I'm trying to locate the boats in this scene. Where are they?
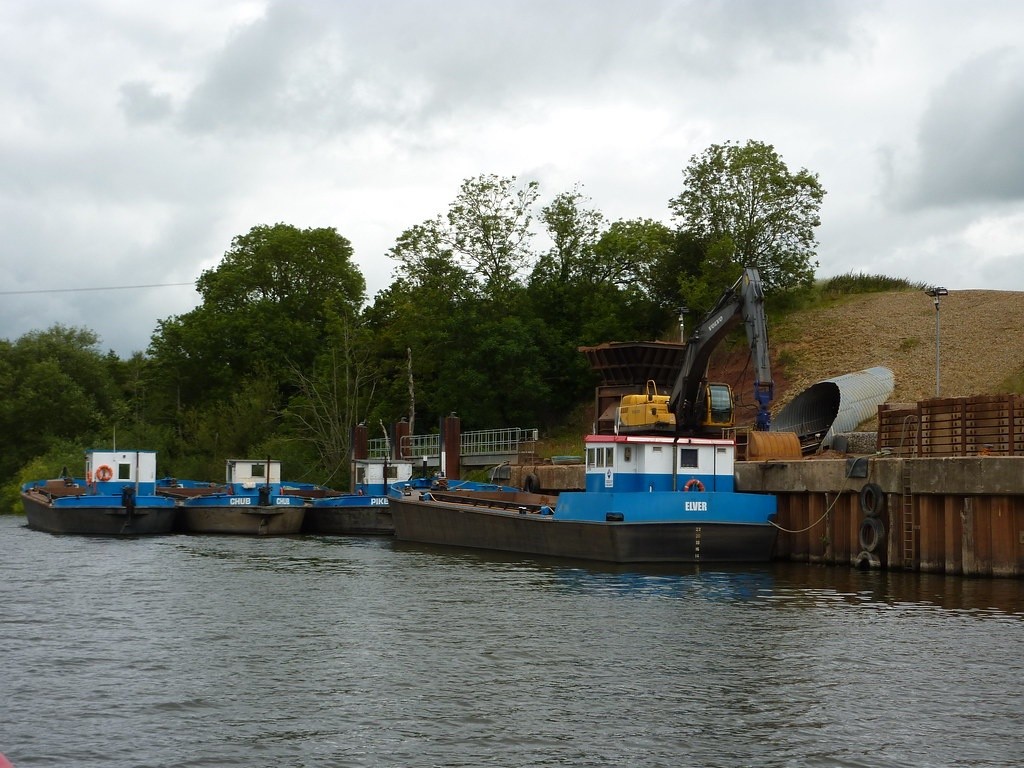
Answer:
[153,479,309,538]
[255,480,397,535]
[386,478,778,567]
[21,476,175,536]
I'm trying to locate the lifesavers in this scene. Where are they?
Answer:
[684,480,704,492]
[96,464,113,481]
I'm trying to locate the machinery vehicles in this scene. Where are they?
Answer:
[614,267,803,463]
